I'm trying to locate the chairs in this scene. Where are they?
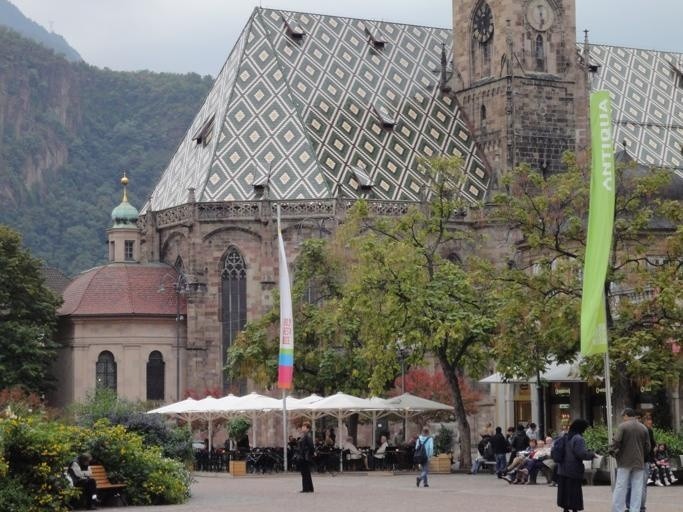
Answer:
[187,447,417,475]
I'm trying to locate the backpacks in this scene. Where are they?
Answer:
[413,436,432,464]
[551,433,567,464]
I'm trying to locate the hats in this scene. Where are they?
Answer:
[78,452,92,467]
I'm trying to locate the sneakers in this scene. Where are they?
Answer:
[416,477,420,487]
[424,484,429,487]
[86,505,97,510]
[655,479,664,487]
[300,489,314,493]
[496,468,538,485]
[647,479,654,484]
[91,499,102,504]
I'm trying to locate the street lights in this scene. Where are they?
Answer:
[529,319,546,440]
[159,269,206,404]
[395,332,408,441]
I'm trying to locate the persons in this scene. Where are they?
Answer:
[475,421,555,486]
[66,453,97,511]
[608,407,678,512]
[555,419,596,511]
[413,427,434,488]
[297,422,313,494]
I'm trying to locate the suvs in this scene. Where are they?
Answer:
[191,438,213,452]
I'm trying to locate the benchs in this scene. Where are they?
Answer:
[614,454,682,483]
[473,457,497,473]
[545,469,597,486]
[62,465,128,506]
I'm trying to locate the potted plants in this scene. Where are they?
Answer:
[427,425,455,475]
[226,415,253,477]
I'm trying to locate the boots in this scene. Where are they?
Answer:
[664,477,671,486]
[670,475,678,483]
